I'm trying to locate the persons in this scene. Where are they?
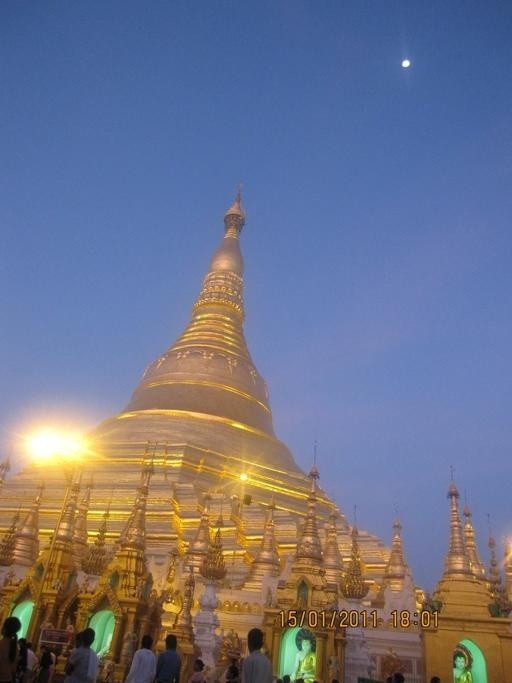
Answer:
[0,616,22,683]
[291,635,317,683]
[48,648,57,683]
[124,635,158,683]
[453,652,473,683]
[224,657,239,683]
[32,645,54,683]
[23,641,39,683]
[64,631,83,675]
[156,634,181,683]
[15,638,28,683]
[187,659,205,683]
[241,628,273,683]
[63,628,97,683]
[276,672,441,683]
